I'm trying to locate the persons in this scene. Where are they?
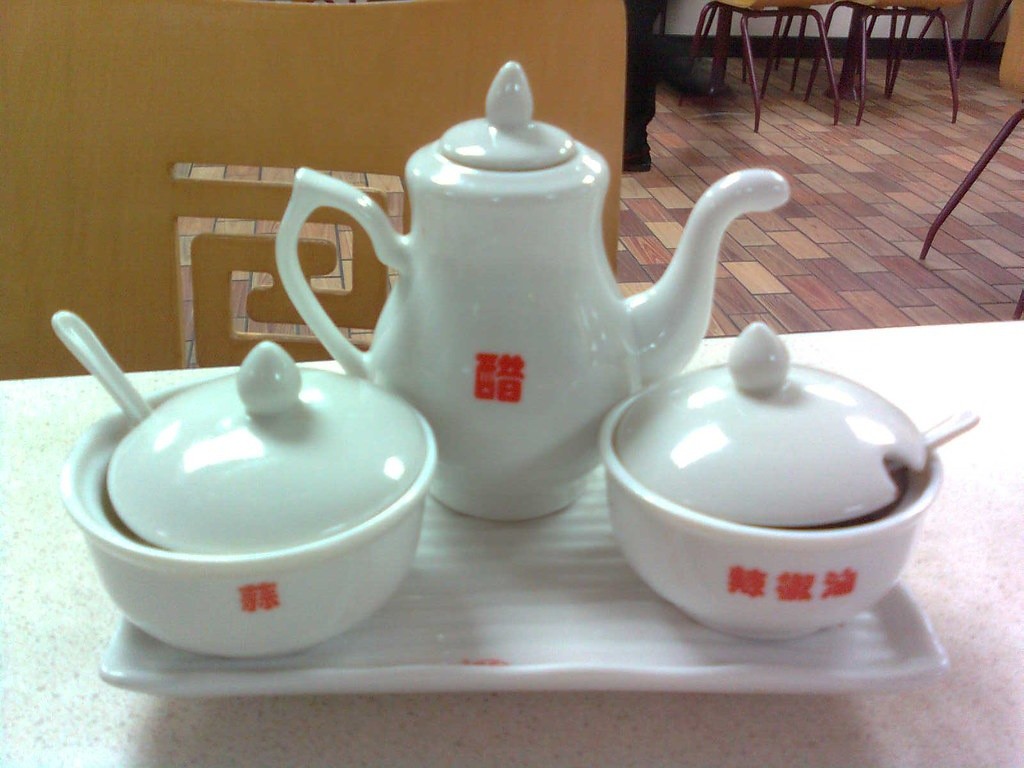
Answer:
[621,0,667,172]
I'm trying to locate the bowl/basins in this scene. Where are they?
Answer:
[62,336,435,663]
[597,322,942,638]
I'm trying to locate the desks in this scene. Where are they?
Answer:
[0,317,1024,768]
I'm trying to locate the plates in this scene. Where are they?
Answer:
[99,468,949,695]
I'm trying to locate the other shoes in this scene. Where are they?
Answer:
[622,153,651,172]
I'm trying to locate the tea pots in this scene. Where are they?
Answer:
[272,60,788,520]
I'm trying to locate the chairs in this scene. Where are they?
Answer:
[802,0,973,126]
[678,0,841,132]
